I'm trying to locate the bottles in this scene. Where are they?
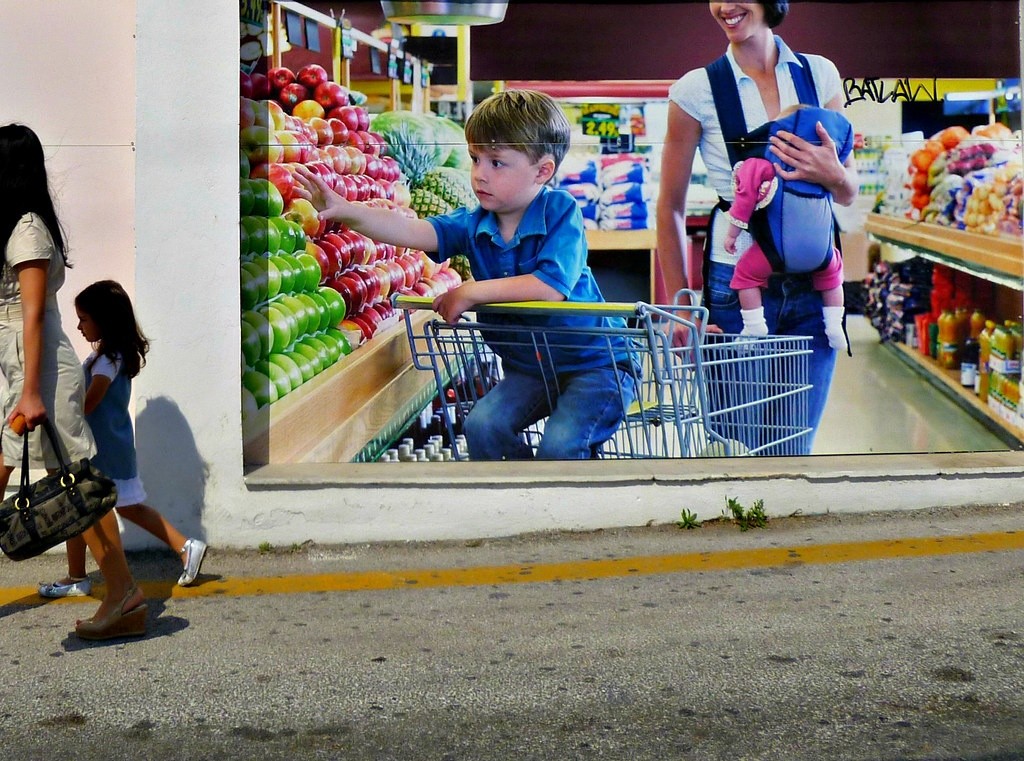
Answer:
[881,136,894,151]
[937,305,1024,430]
[375,369,497,461]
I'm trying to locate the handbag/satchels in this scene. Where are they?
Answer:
[1,418,118,562]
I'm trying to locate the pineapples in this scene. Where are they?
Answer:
[382,122,480,282]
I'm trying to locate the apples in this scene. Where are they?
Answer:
[240,65,462,417]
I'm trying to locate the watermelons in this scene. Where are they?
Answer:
[369,110,472,168]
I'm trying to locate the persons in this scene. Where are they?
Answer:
[0,126,151,640]
[292,90,642,460]
[37,281,207,596]
[725,106,848,350]
[655,1,860,457]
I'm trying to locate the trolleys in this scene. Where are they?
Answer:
[387,286,814,462]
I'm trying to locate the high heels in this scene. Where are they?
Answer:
[74,584,148,640]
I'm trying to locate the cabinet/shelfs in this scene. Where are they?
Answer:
[864,211,1024,450]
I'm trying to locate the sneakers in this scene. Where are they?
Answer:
[177,538,208,586]
[38,575,92,597]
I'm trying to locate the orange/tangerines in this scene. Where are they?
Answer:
[908,123,1009,208]
